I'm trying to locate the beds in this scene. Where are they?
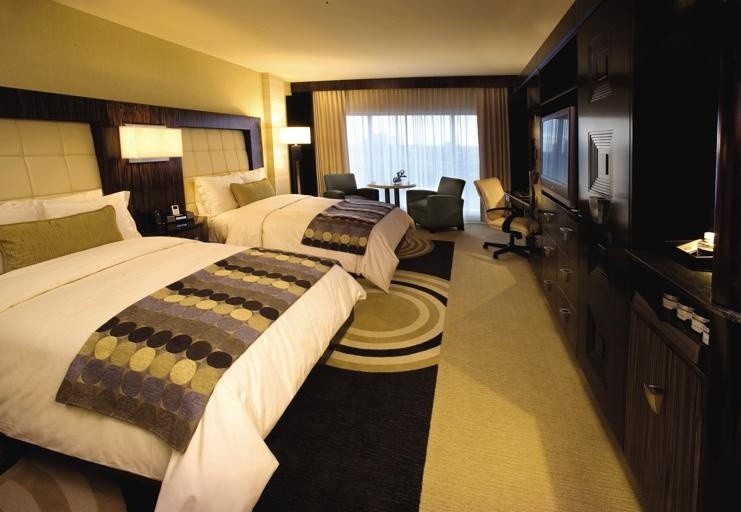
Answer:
[197,195,409,279]
[0,235,356,501]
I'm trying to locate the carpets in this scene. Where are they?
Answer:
[0,235,455,512]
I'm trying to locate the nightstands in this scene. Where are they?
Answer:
[137,211,208,243]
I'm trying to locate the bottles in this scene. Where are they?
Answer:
[696,232,714,255]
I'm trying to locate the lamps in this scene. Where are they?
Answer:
[276,126,311,193]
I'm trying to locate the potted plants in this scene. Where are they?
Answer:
[392,168,406,186]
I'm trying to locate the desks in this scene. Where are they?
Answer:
[504,189,529,216]
[366,181,415,209]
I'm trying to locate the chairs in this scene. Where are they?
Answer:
[405,176,466,233]
[321,173,378,203]
[473,176,530,263]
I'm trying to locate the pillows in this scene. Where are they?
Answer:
[193,166,275,217]
[0,189,142,276]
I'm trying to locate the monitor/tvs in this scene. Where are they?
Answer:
[541,105,577,209]
